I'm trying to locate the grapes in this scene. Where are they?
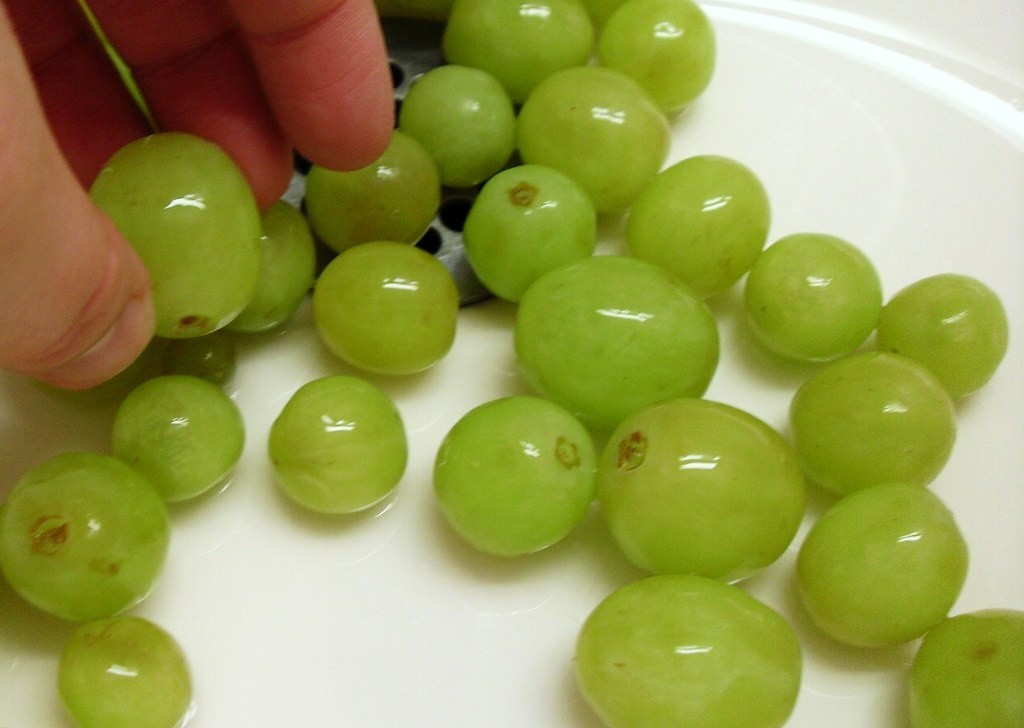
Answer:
[0,0,1024,728]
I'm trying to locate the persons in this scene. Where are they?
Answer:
[0,0,394,393]
